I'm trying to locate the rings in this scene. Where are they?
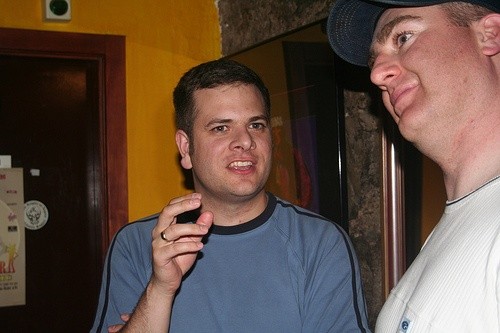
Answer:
[161,232,171,242]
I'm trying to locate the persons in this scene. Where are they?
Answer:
[322,0,500,333]
[89,59,372,333]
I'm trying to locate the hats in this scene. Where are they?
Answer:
[327,0,500,66]
